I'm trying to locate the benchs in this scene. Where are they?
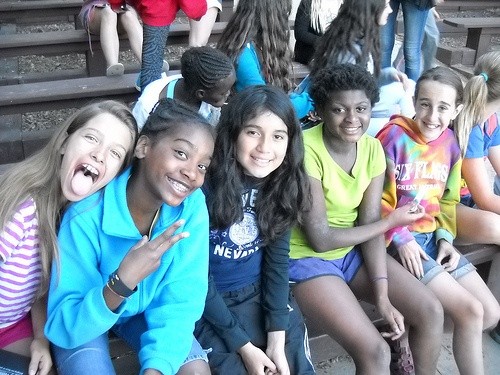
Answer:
[0,0,500,375]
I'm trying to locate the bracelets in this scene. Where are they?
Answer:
[105,270,138,300]
[370,275,390,284]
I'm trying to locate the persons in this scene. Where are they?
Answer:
[372,66,500,375]
[0,100,139,375]
[42,96,212,375]
[447,50,500,345]
[76,0,442,139]
[193,85,317,375]
[288,63,445,375]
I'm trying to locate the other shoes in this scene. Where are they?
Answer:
[105,62,125,76]
[134,71,142,92]
[391,346,415,375]
[161,60,170,73]
